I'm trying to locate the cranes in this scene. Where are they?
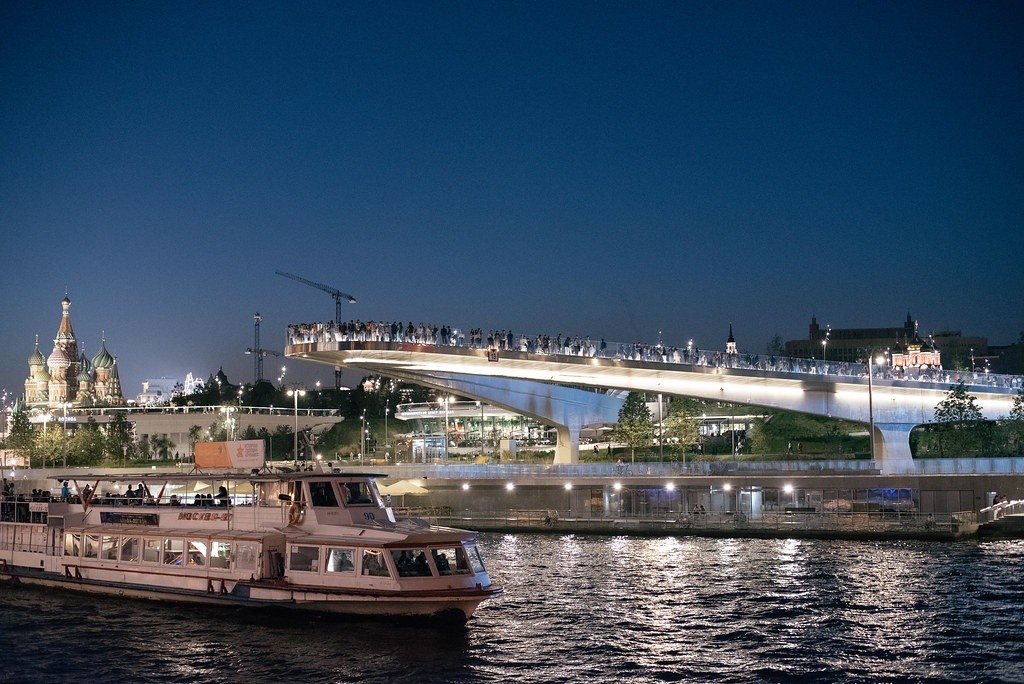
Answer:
[274,268,358,391]
[247,346,281,382]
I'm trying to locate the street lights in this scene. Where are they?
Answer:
[822,341,826,362]
[505,483,514,523]
[438,396,455,462]
[666,483,674,519]
[287,389,306,460]
[564,483,572,518]
[724,484,731,515]
[614,483,622,520]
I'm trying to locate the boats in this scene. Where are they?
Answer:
[0,436,503,633]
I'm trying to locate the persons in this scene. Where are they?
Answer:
[993,495,1008,520]
[166,551,230,568]
[544,511,559,527]
[0,478,231,506]
[693,503,706,521]
[928,512,935,522]
[616,458,624,466]
[287,320,1024,389]
[294,460,341,473]
[593,444,611,457]
[336,547,450,576]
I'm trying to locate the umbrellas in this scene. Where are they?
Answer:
[360,479,434,507]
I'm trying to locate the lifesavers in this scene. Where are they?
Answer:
[289,504,301,525]
